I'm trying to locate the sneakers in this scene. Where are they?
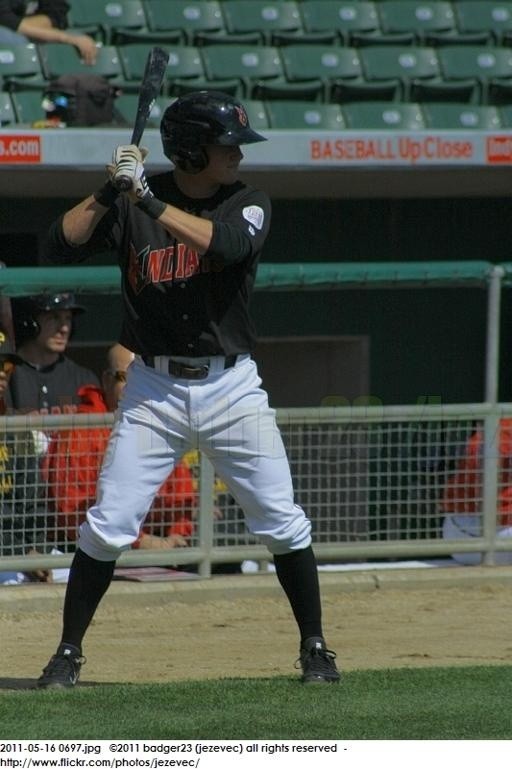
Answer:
[36,642,87,688]
[294,637,341,683]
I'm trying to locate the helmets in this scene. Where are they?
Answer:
[160,90,268,175]
[13,291,87,340]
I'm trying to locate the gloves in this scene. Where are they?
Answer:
[106,144,155,210]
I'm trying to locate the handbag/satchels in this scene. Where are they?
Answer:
[46,72,128,128]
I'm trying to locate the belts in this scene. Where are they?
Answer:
[142,351,237,380]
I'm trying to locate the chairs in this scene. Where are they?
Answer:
[0,0,511,128]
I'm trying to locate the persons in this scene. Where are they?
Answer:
[0,0,99,67]
[0,331,74,584]
[6,292,100,456]
[40,91,339,690]
[44,342,241,573]
[443,419,510,564]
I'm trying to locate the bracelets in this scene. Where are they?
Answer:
[140,196,166,219]
[91,178,118,205]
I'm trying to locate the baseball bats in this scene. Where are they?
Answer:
[114,49,169,191]
